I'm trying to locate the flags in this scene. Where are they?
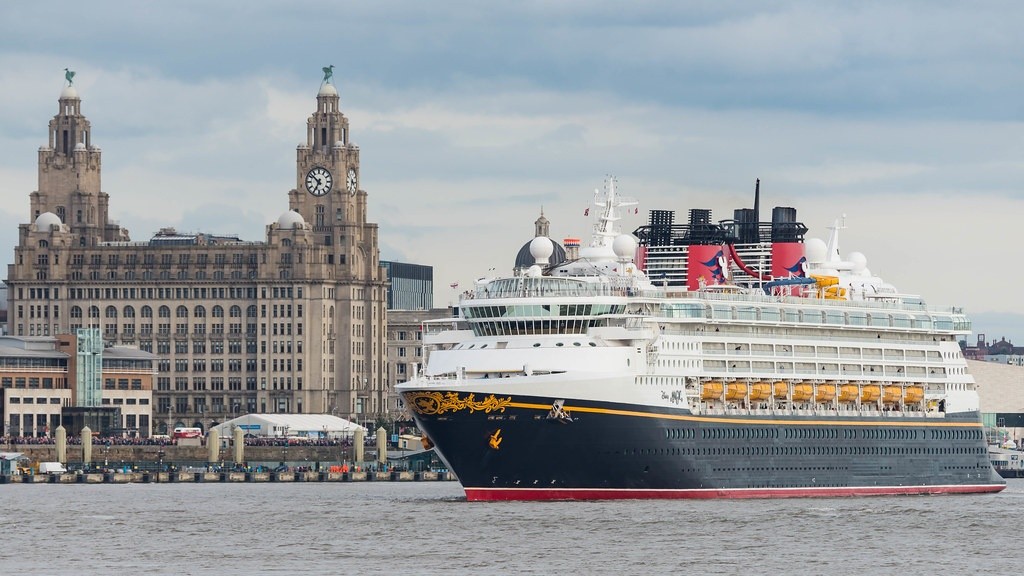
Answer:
[635,207,638,214]
[584,208,589,216]
[420,324,423,330]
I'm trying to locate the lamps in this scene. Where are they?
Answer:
[612,234,636,259]
[804,237,827,267]
[530,235,553,266]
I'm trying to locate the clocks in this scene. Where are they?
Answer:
[345,168,358,197]
[304,167,332,197]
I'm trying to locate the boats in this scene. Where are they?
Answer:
[774,382,788,399]
[883,386,901,404]
[725,382,747,400]
[699,380,723,399]
[792,382,814,400]
[814,385,835,402]
[903,387,923,404]
[861,386,880,402]
[750,382,771,400]
[838,386,859,402]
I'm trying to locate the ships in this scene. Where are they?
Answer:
[393,173,1010,498]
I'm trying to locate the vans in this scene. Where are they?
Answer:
[39,462,68,475]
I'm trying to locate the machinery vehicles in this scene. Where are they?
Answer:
[16,457,33,475]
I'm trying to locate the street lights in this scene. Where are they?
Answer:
[342,427,348,465]
[166,405,173,435]
[233,404,238,428]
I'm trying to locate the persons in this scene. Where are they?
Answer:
[0,435,177,446]
[208,463,263,472]
[363,438,377,447]
[229,435,354,447]
[297,464,412,474]
